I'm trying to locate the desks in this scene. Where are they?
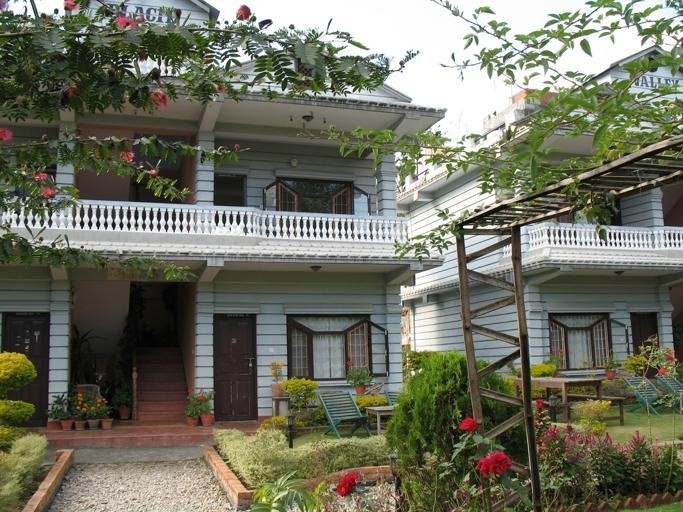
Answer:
[510,373,607,426]
[361,405,395,434]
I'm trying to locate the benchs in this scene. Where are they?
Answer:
[533,397,568,424]
[552,391,625,428]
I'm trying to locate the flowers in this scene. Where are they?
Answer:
[266,360,286,384]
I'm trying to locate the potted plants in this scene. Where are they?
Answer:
[602,350,618,381]
[344,366,374,396]
[196,387,216,427]
[182,391,199,427]
[41,375,132,431]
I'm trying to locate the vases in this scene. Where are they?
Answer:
[269,382,284,397]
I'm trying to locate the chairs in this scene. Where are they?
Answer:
[621,375,680,416]
[316,390,373,443]
[653,373,683,415]
[383,388,402,406]
